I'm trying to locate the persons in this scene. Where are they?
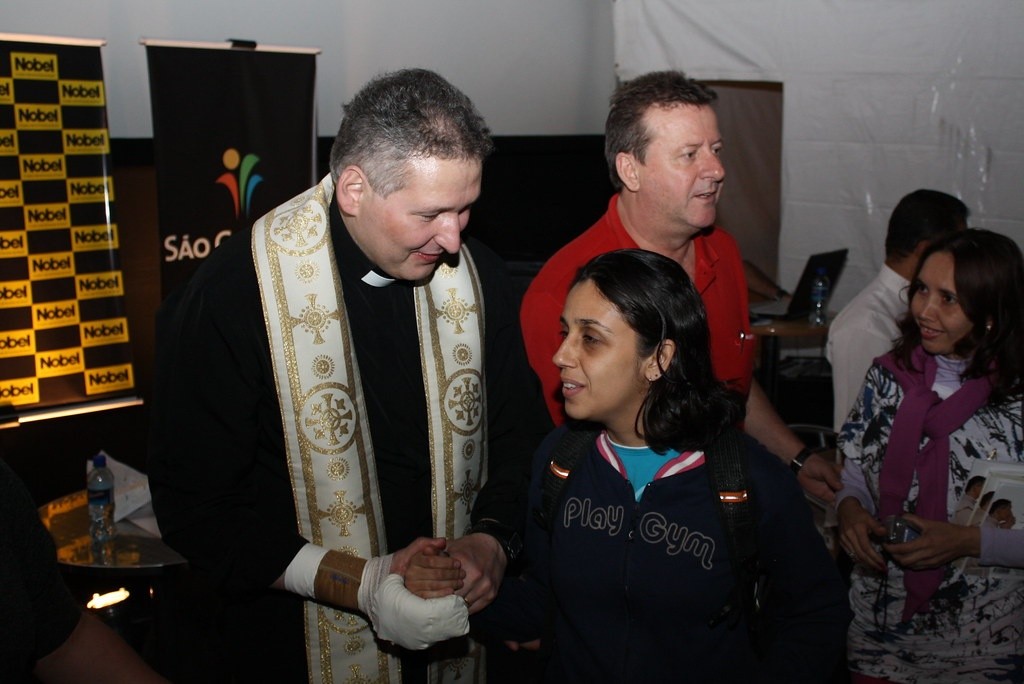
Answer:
[985,498,1017,531]
[741,259,791,318]
[404,247,838,683]
[155,62,541,684]
[972,490,995,528]
[0,459,170,684]
[824,188,971,433]
[830,228,1024,683]
[955,476,985,527]
[518,66,845,506]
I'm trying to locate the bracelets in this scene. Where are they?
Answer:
[773,290,785,301]
[790,448,813,476]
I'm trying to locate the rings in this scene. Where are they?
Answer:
[463,597,471,606]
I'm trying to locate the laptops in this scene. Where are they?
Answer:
[749,248,849,320]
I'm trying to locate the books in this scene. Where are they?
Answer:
[946,460,1024,582]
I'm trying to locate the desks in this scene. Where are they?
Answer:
[36,493,191,621]
[752,310,839,408]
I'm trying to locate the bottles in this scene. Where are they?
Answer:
[85,455,118,540]
[808,269,831,326]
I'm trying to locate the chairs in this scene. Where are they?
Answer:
[787,423,842,564]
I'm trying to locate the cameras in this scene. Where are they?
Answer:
[886,513,921,545]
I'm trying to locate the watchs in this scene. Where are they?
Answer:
[467,519,524,559]
